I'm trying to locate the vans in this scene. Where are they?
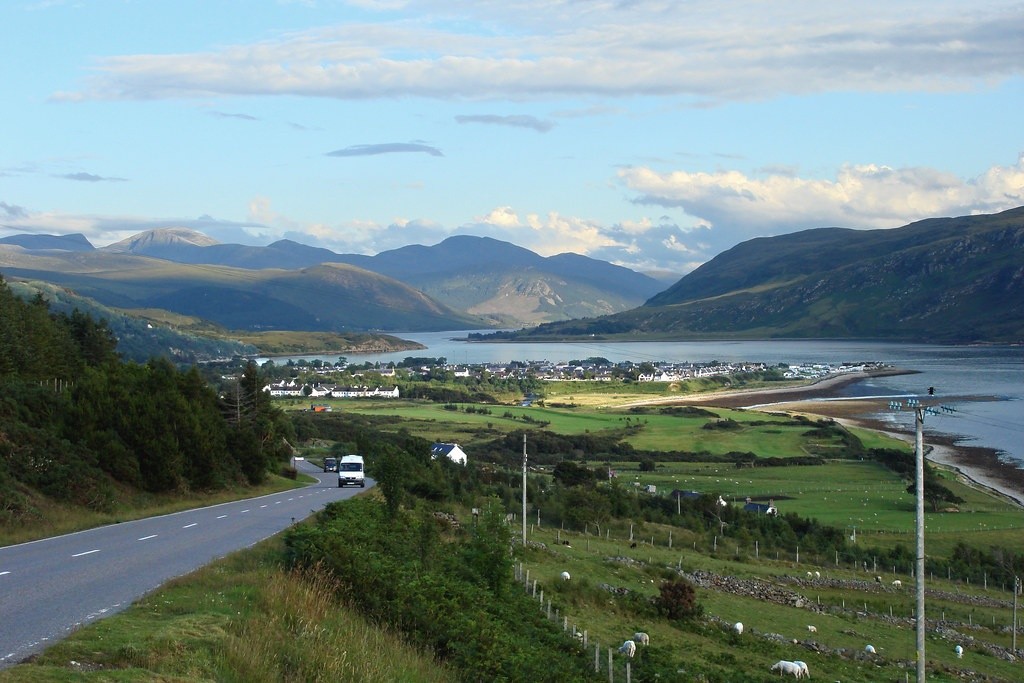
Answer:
[324,458,337,473]
[337,454,366,488]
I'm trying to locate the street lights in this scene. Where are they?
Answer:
[884,399,958,683]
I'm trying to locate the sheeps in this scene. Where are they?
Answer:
[561,544,963,681]
